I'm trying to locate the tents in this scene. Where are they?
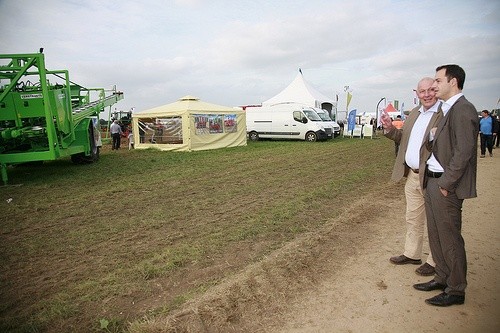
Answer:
[261,68,336,123]
[132,96,246,152]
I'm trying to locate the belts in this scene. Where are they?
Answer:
[426,168,443,178]
[408,165,419,173]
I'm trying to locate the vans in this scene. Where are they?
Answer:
[312,108,340,139]
[245,107,334,142]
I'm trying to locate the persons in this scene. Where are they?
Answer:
[138,119,164,143]
[479,110,500,157]
[110,120,124,152]
[380,77,445,277]
[394,115,403,158]
[412,64,480,308]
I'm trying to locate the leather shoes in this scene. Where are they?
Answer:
[390,254,422,265]
[413,279,448,292]
[415,262,436,276]
[425,292,465,307]
